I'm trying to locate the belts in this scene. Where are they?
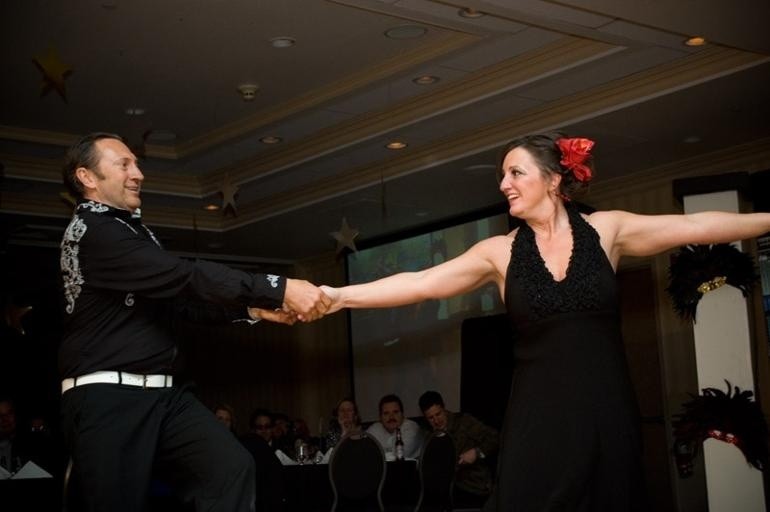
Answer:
[62,371,173,394]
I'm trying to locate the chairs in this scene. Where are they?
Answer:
[326,431,458,512]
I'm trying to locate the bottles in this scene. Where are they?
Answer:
[395,428,405,461]
